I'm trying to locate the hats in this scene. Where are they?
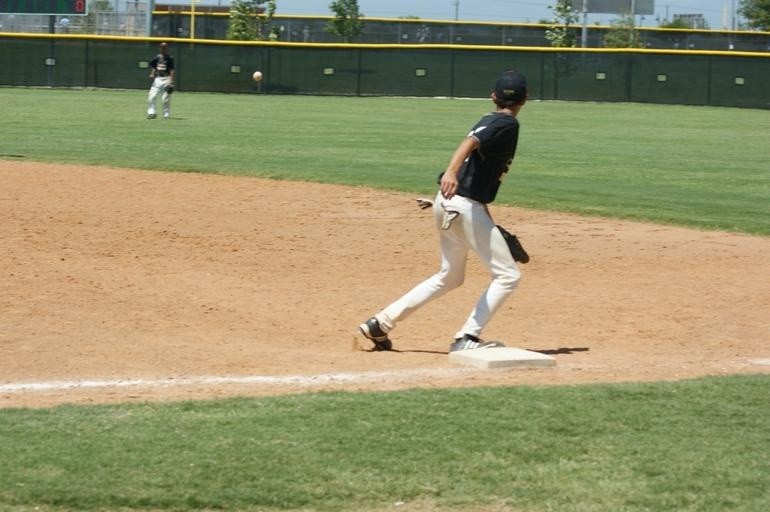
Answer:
[494,69,526,101]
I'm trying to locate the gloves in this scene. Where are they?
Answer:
[416,199,433,210]
[441,211,458,230]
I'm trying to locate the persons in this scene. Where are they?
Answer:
[357,71,528,352]
[146,42,175,119]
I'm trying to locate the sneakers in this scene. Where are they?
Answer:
[146,114,155,119]
[359,317,392,351]
[449,333,504,353]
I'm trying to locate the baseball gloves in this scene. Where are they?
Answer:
[495,223,529,264]
[164,81,175,92]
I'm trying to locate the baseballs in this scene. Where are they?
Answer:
[253,72,263,82]
